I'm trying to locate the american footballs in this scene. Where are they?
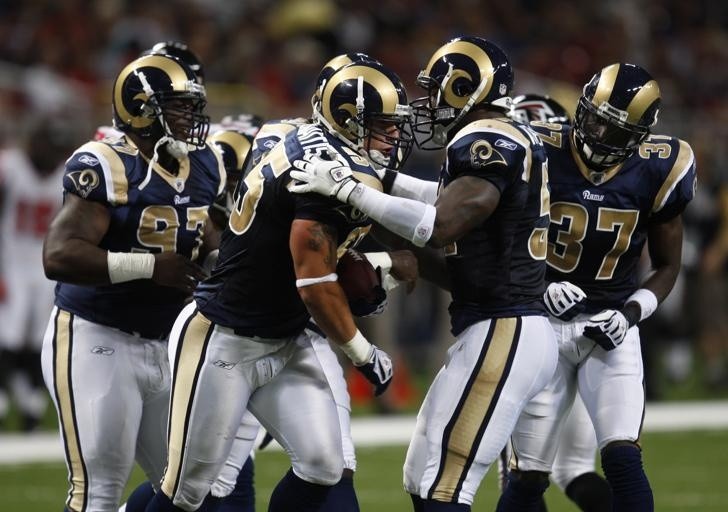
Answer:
[336,247,380,307]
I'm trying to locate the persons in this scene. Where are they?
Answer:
[288,35,698,512]
[35,42,416,512]
[0,1,727,418]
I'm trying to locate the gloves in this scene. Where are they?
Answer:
[582,308,629,351]
[351,343,393,398]
[542,281,587,321]
[288,144,360,204]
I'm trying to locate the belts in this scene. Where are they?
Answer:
[119,329,168,340]
[233,321,326,339]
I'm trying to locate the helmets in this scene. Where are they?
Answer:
[152,40,205,85]
[406,35,514,150]
[112,53,209,147]
[311,51,378,120]
[312,60,415,173]
[220,112,264,138]
[571,61,661,171]
[507,93,571,126]
